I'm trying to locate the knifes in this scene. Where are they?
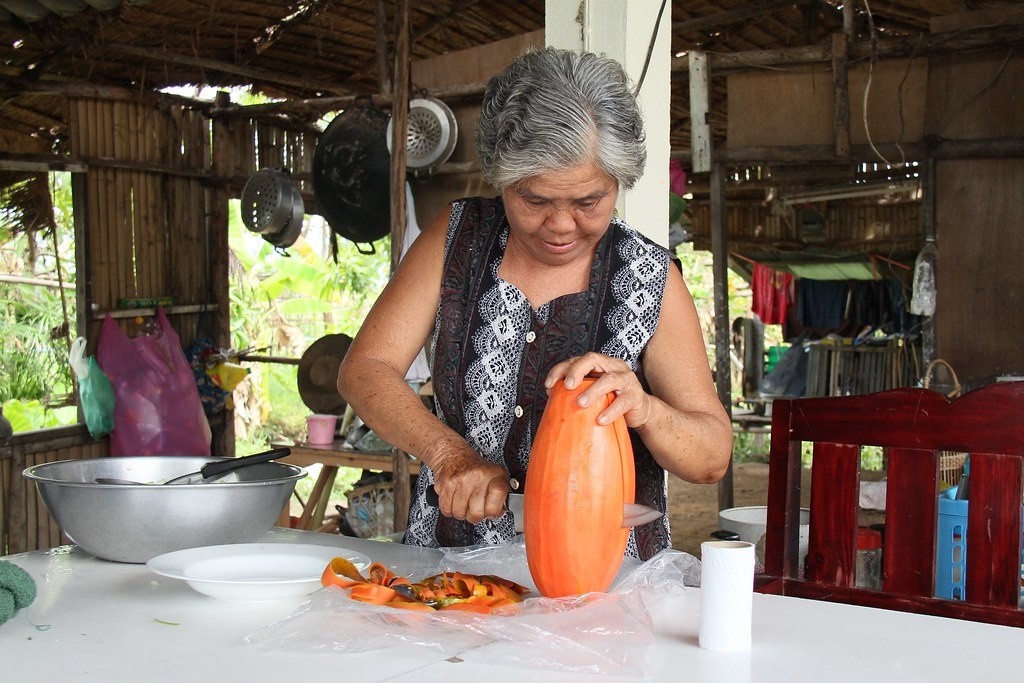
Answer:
[424,483,664,533]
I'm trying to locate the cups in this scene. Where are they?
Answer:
[699,540,757,652]
[303,414,338,446]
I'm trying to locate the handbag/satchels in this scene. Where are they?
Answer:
[67,336,89,382]
[98,303,213,457]
[184,303,233,421]
[70,355,117,439]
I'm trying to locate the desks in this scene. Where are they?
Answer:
[271,439,422,539]
[0,526,1024,683]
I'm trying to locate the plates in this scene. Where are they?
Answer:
[145,542,371,602]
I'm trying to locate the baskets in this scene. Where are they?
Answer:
[925,359,968,489]
[344,482,395,539]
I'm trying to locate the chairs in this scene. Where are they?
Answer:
[757,382,1024,629]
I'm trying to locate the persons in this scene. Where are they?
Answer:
[337,46,733,561]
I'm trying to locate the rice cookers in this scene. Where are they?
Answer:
[710,504,812,573]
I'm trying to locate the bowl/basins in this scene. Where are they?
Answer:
[21,454,307,565]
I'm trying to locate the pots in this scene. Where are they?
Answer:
[313,93,392,256]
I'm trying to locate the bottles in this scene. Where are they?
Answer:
[911,234,937,316]
[135,316,157,334]
[204,361,250,393]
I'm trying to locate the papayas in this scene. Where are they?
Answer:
[522,378,637,601]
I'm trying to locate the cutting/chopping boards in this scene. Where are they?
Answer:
[297,333,353,415]
